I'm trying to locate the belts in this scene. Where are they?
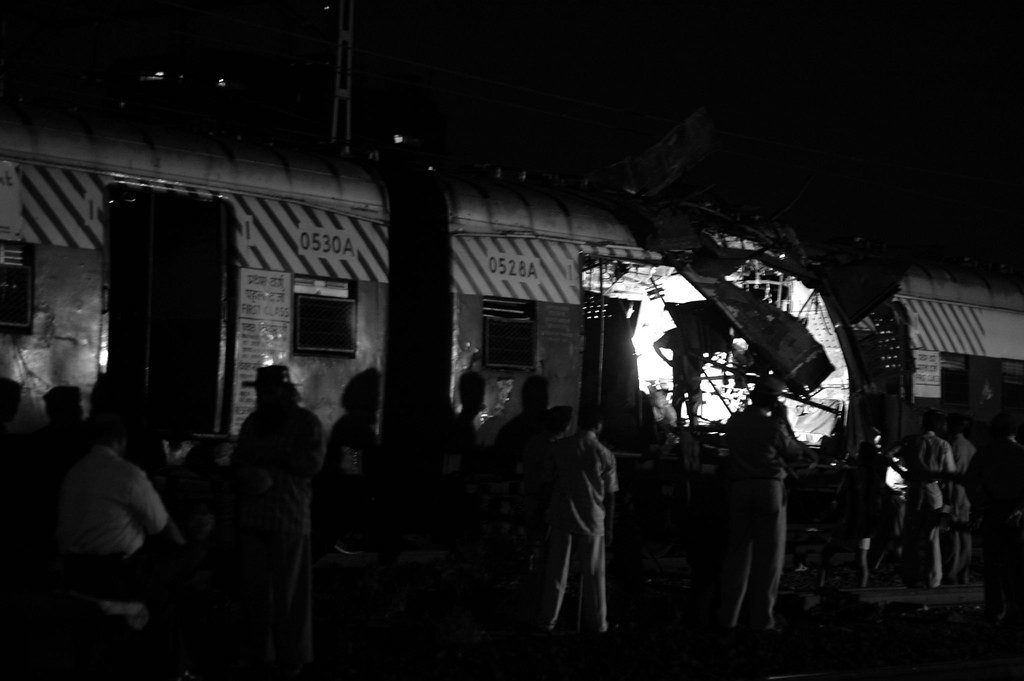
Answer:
[731,476,782,481]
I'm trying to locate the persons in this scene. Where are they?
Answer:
[653,326,702,426]
[538,402,620,633]
[54,365,1023,681]
